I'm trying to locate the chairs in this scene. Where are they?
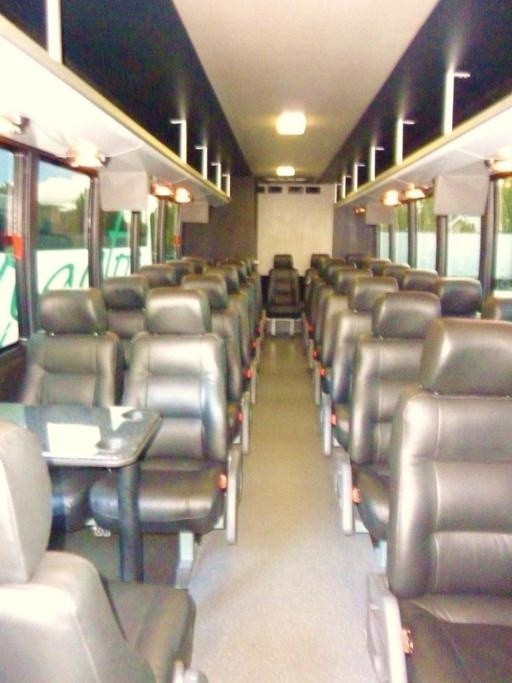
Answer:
[262,253,305,337]
[366,318,512,683]
[0,413,195,683]
[298,252,512,568]
[0,252,262,590]
